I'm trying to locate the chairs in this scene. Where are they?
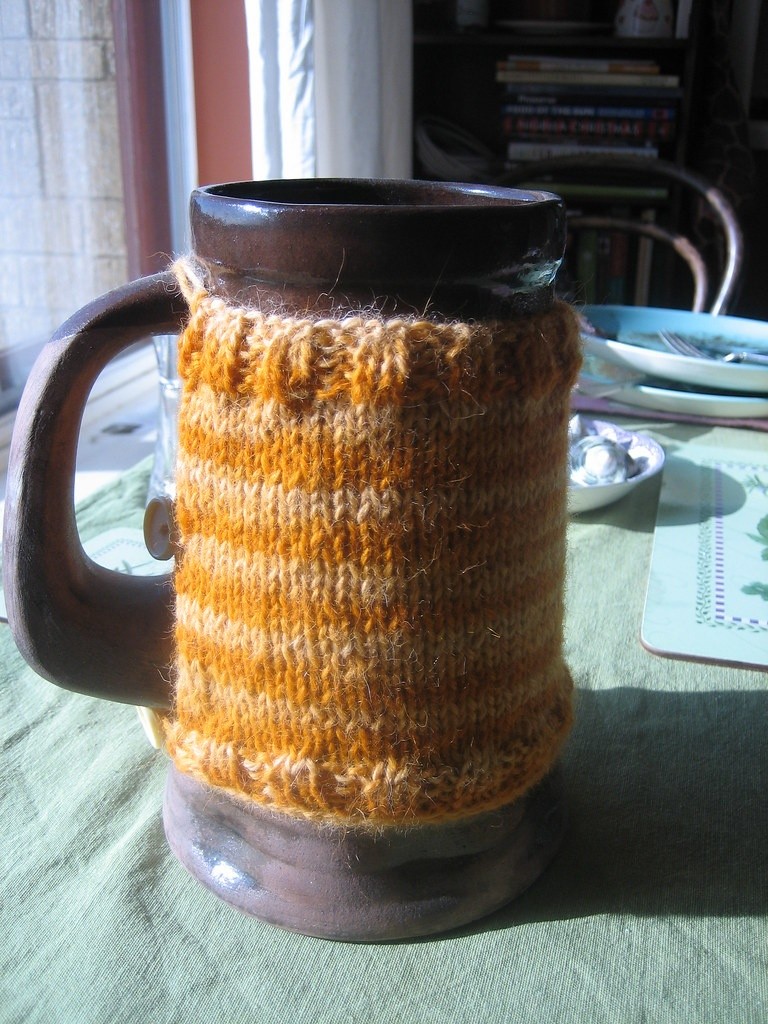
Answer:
[497,152,744,319]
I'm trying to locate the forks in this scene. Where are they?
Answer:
[656,330,768,369]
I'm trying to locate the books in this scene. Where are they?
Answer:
[496,54,684,304]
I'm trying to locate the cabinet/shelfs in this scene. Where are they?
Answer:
[412,30,709,307]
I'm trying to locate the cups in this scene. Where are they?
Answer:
[0,177,575,943]
[520,0,573,21]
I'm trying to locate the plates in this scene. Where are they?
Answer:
[492,20,605,34]
[574,305,768,417]
[569,413,665,513]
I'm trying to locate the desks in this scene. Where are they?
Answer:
[0,351,767,1024]
